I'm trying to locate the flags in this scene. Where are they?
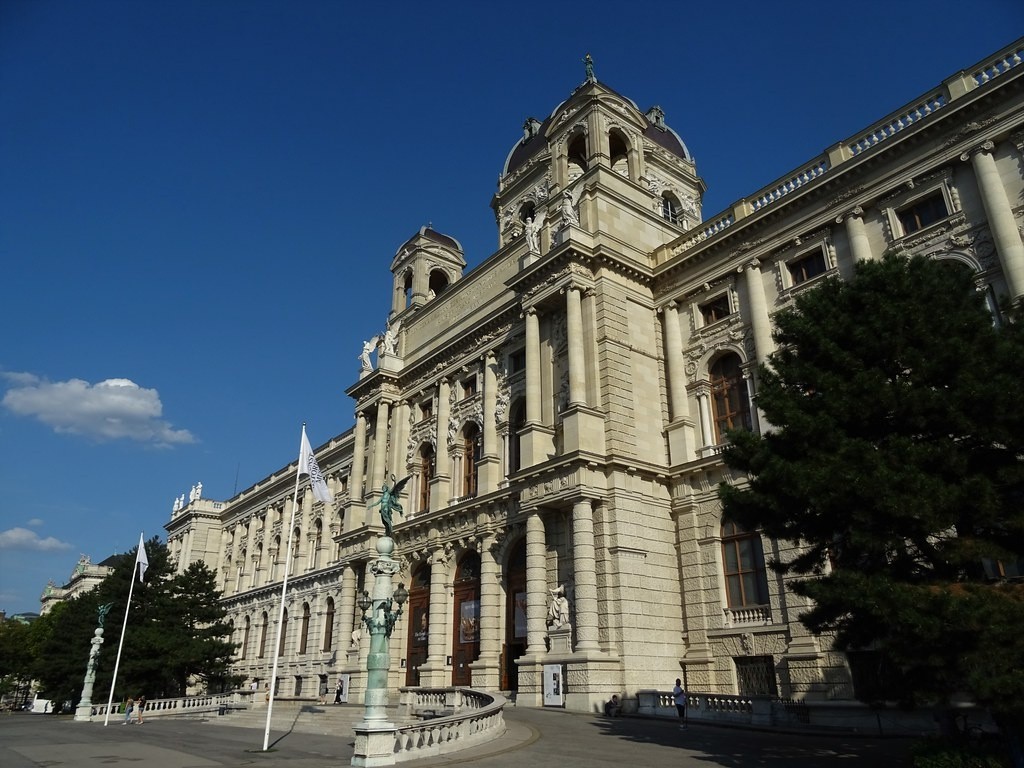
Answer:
[297,426,335,503]
[137,533,149,582]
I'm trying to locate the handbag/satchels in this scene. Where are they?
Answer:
[325,688,328,694]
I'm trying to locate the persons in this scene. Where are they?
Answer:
[189,485,195,503]
[383,323,396,357]
[367,485,394,536]
[173,497,179,513]
[135,695,147,724]
[605,695,618,717]
[673,678,690,729]
[557,189,579,226]
[265,682,269,702]
[251,680,258,690]
[933,703,969,740]
[122,695,134,726]
[195,481,202,499]
[519,212,537,251]
[333,680,343,705]
[318,673,328,706]
[178,494,185,510]
[358,340,373,372]
[550,592,570,627]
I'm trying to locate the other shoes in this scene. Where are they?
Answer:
[129,720,133,723]
[680,725,689,731]
[333,702,335,705]
[135,722,139,724]
[122,723,127,725]
[339,702,342,705]
[139,722,143,724]
